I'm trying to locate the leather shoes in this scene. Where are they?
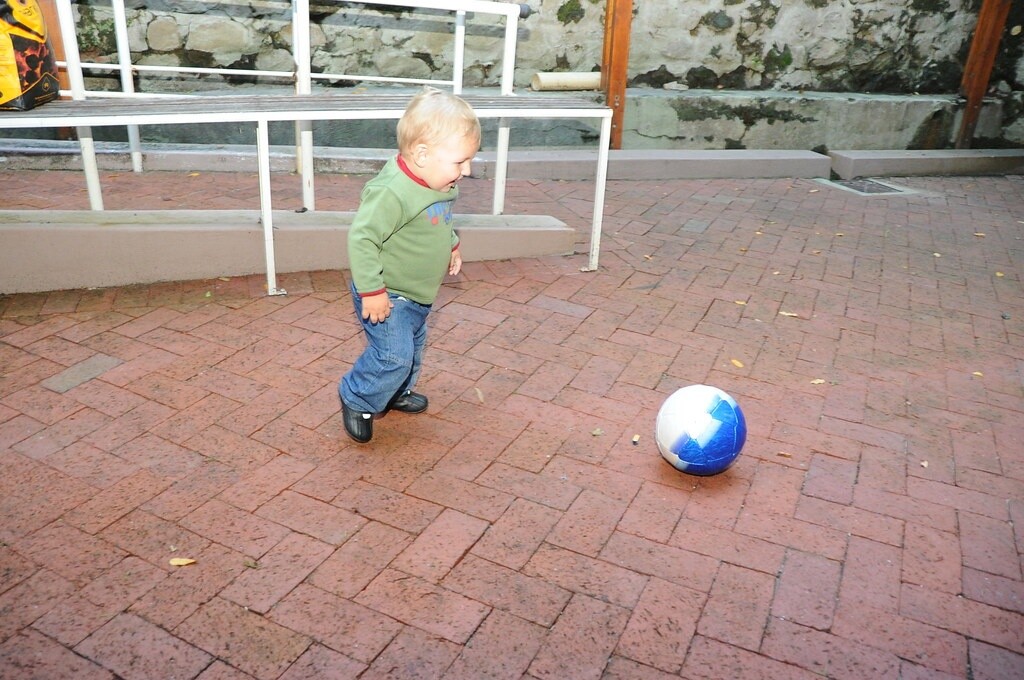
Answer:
[386,390,428,413]
[339,393,372,443]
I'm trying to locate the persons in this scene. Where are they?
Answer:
[339,91,481,443]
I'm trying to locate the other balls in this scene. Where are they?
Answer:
[655,383,746,478]
